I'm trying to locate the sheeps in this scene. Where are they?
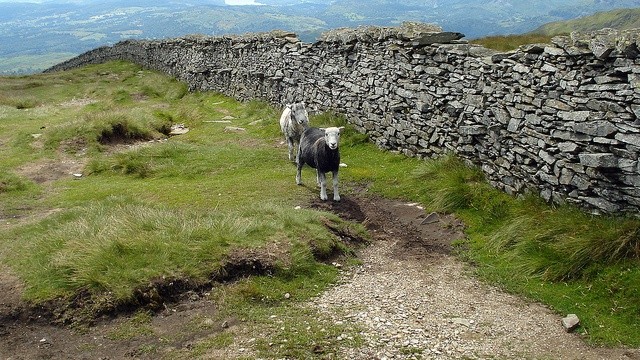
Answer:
[295,125,345,201]
[279,100,309,160]
